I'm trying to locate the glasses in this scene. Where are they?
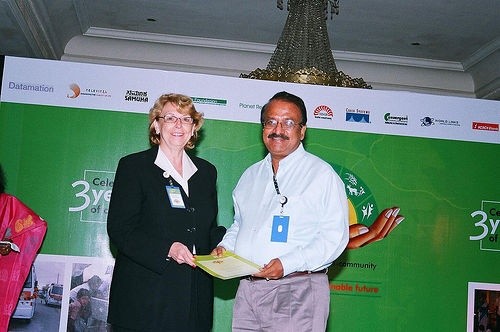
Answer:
[263,119,302,129]
[156,115,195,124]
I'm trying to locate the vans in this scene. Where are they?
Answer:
[38,283,63,307]
[11,264,38,319]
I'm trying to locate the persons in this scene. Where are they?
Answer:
[210,91,350,332]
[67,275,102,332]
[106,92,227,332]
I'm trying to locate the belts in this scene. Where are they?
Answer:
[242,269,326,280]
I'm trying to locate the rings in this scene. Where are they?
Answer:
[266,277,269,281]
